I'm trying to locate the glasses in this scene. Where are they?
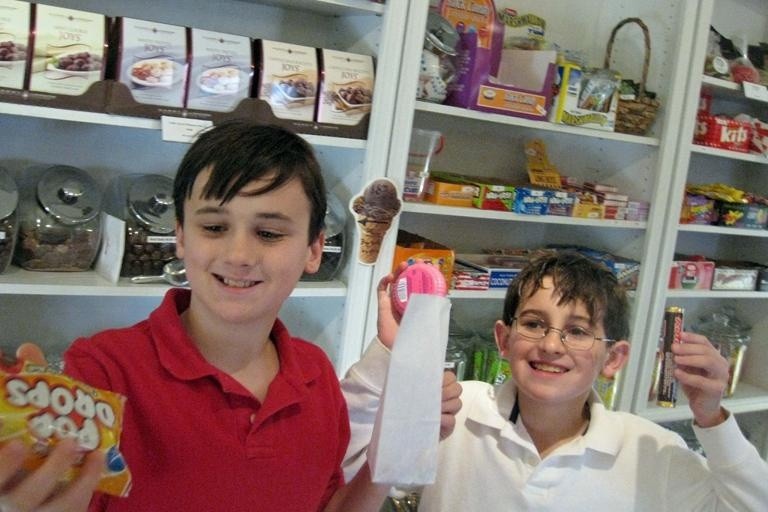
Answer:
[511,314,618,352]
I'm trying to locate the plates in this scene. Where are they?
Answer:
[0,41,254,95]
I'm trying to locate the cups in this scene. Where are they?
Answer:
[402,128,443,203]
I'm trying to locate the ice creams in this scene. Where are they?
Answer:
[350,179,402,264]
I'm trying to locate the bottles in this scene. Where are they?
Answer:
[415,8,463,104]
[689,305,752,399]
[13,163,103,273]
[298,190,347,282]
[105,172,177,278]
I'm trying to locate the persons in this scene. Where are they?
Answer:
[337,257,768,512]
[0,117,462,512]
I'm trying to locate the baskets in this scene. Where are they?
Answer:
[602,17,661,136]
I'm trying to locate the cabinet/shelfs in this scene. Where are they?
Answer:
[1,0,768,509]
